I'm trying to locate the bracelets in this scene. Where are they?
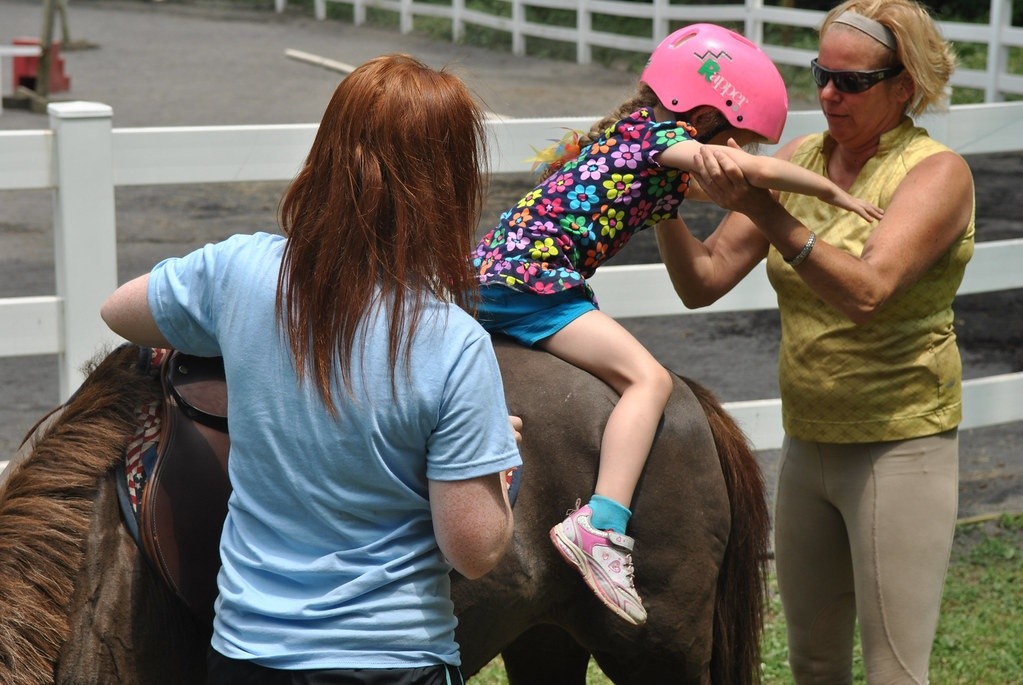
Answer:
[786,229,819,266]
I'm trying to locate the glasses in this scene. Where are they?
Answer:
[810,58,905,93]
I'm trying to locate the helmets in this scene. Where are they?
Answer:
[640,22,789,144]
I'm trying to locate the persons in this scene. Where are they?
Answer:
[654,1,981,685]
[99,51,524,685]
[462,21,886,624]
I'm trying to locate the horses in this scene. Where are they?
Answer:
[0,340,776,685]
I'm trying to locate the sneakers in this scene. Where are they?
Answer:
[548,503,649,626]
